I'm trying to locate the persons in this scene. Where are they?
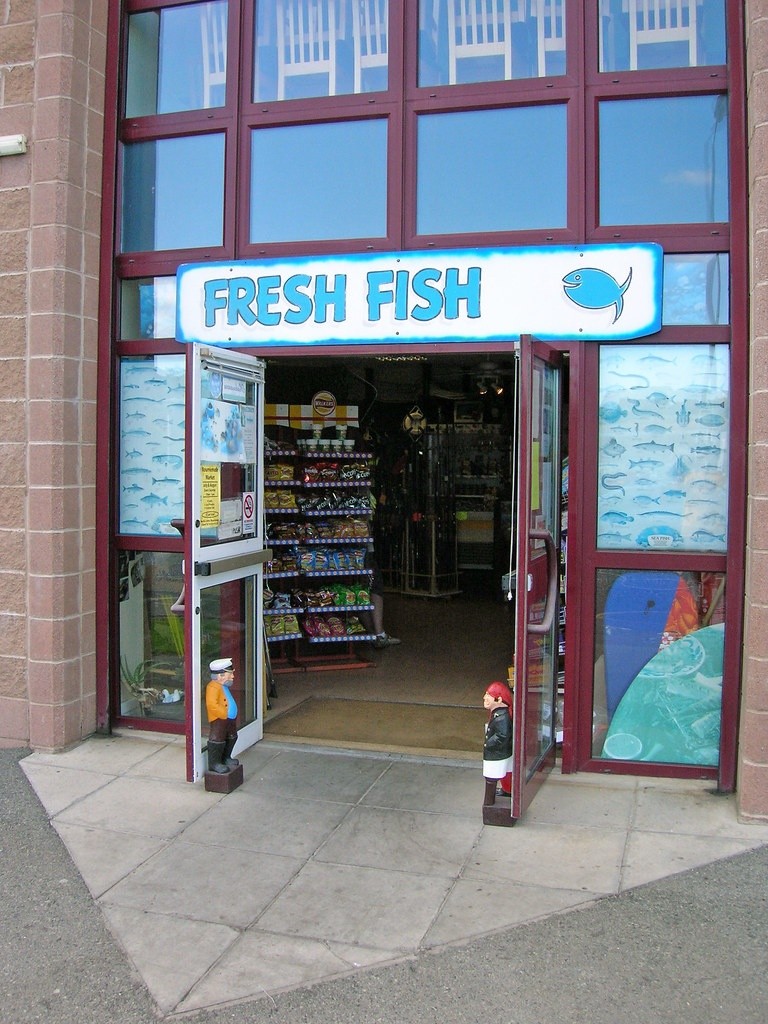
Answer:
[482,681,514,798]
[358,492,401,647]
[206,658,239,773]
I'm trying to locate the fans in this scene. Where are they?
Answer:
[446,354,515,377]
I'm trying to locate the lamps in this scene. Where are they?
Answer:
[492,378,503,394]
[476,378,486,395]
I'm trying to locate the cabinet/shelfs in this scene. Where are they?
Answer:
[299,452,380,642]
[264,449,303,642]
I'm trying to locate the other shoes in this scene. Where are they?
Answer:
[374,631,401,648]
